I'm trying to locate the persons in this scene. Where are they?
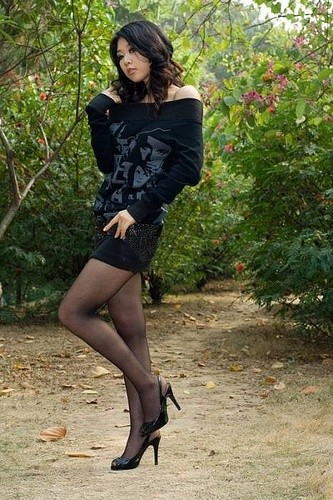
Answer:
[57,21,204,470]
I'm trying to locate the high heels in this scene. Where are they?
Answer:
[110,433,161,470]
[141,375,181,435]
